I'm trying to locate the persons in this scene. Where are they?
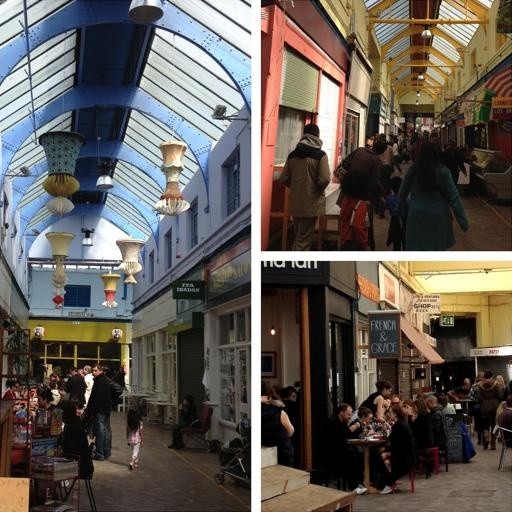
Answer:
[1,363,145,479]
[278,122,485,250]
[167,394,197,449]
[261,369,512,495]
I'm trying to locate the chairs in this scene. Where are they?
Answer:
[497,425,512,471]
[180,406,214,448]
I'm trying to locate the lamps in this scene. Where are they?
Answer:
[5,166,32,177]
[154,32,191,215]
[95,104,114,189]
[128,0,164,23]
[271,287,276,335]
[100,263,121,307]
[116,201,145,284]
[38,30,86,215]
[45,213,76,309]
[80,199,95,246]
[415,22,432,106]
[211,103,249,121]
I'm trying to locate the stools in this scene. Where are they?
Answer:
[65,477,97,512]
[322,468,351,490]
[34,479,68,503]
[392,443,449,494]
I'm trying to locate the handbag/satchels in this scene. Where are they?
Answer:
[333,163,348,179]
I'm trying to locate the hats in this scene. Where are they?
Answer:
[303,123,319,138]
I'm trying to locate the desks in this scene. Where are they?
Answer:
[118,390,177,431]
[0,400,13,476]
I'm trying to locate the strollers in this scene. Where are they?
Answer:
[214,418,251,486]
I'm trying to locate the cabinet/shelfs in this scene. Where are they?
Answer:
[470,147,512,199]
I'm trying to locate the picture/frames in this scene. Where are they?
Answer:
[261,351,277,379]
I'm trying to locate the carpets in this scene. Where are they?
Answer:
[346,436,387,494]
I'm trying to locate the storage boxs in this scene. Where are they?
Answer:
[32,460,79,482]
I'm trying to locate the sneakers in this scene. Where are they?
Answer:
[129,461,141,470]
[355,484,394,497]
[168,441,184,450]
[93,453,111,461]
